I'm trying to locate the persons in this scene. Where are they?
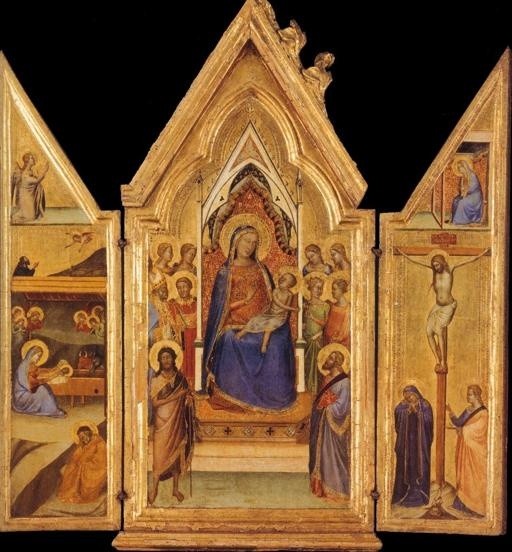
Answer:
[59,425,108,504]
[451,159,484,223]
[395,245,488,374]
[65,231,95,252]
[15,306,105,418]
[147,239,201,394]
[14,255,38,276]
[203,225,297,415]
[234,271,295,354]
[307,349,350,505]
[300,238,351,394]
[11,152,48,223]
[146,349,194,507]
[393,383,435,508]
[445,384,486,519]
